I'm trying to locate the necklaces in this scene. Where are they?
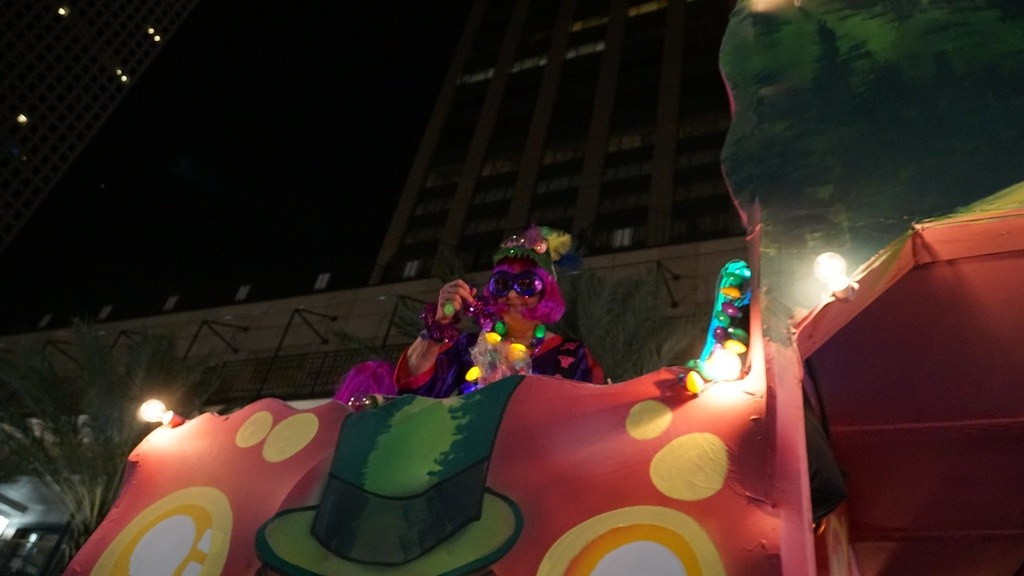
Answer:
[530,336,543,359]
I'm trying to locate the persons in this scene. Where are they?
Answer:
[392,226,610,399]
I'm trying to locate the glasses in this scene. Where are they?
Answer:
[488,270,543,297]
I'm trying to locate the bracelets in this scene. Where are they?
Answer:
[418,304,463,343]
